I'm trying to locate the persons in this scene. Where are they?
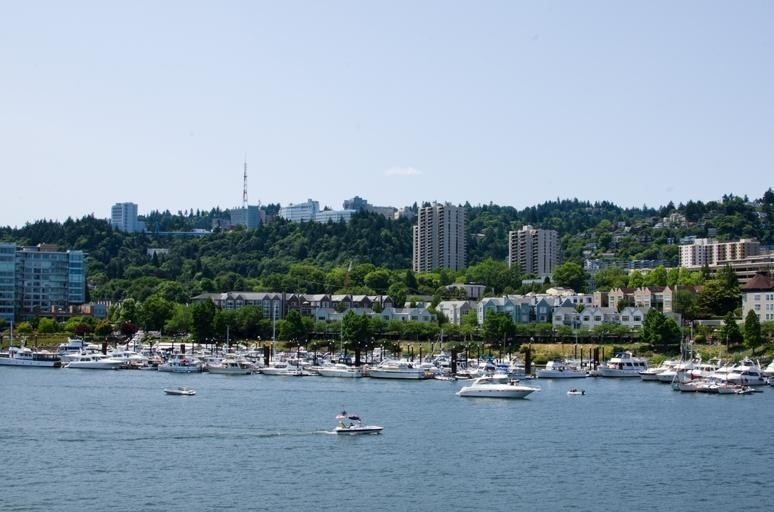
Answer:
[339,421,353,429]
[569,388,578,392]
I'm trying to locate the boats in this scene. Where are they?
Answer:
[304,363,360,378]
[65,343,316,376]
[455,374,540,398]
[164,385,196,396]
[368,353,536,380]
[3,346,62,368]
[637,356,772,393]
[538,360,586,379]
[333,423,385,437]
[596,351,648,377]
